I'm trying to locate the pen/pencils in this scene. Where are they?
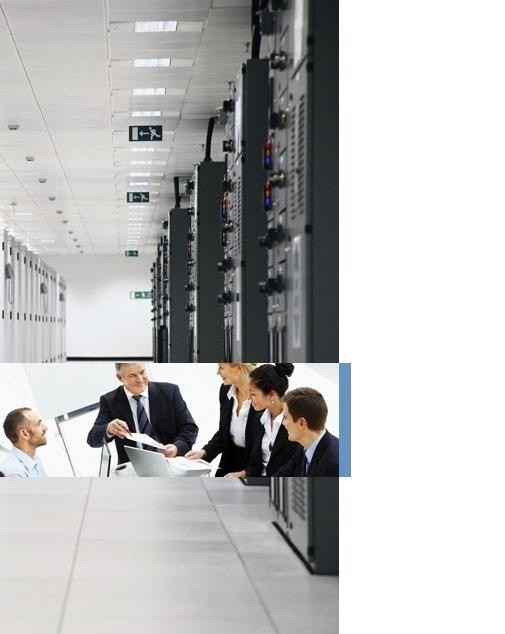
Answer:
[122,426,133,438]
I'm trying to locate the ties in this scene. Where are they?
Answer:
[300,452,309,477]
[131,394,162,452]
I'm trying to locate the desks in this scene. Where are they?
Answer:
[113,457,210,479]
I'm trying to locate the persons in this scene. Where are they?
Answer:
[185,363,256,477]
[0,408,48,480]
[87,362,198,470]
[223,362,300,477]
[272,388,340,476]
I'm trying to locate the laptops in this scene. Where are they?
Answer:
[97,438,112,476]
[123,445,211,478]
